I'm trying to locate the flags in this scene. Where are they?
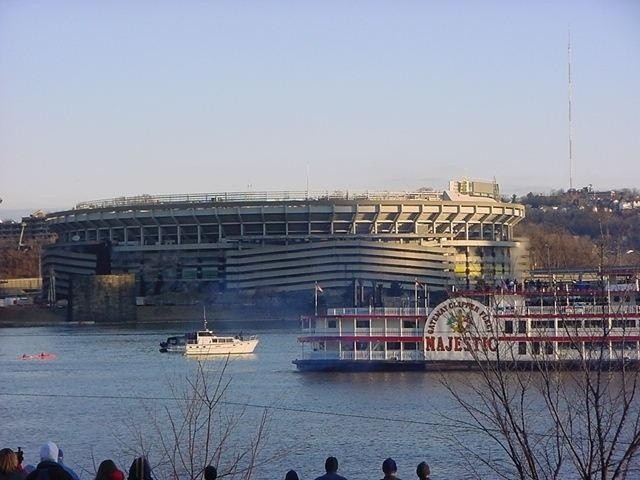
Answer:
[417,280,425,289]
[501,279,510,293]
[317,284,323,293]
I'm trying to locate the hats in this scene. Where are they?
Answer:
[40,441,58,463]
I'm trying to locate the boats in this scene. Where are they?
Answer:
[181,352,258,360]
[159,307,258,354]
[293,241,640,374]
[18,351,57,362]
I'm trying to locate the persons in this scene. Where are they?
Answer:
[514,278,563,292]
[380,458,401,480]
[285,470,299,480]
[417,461,433,480]
[204,466,216,480]
[0,443,152,479]
[315,457,346,480]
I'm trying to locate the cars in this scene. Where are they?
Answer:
[1,288,42,307]
[46,299,68,308]
[135,295,256,307]
